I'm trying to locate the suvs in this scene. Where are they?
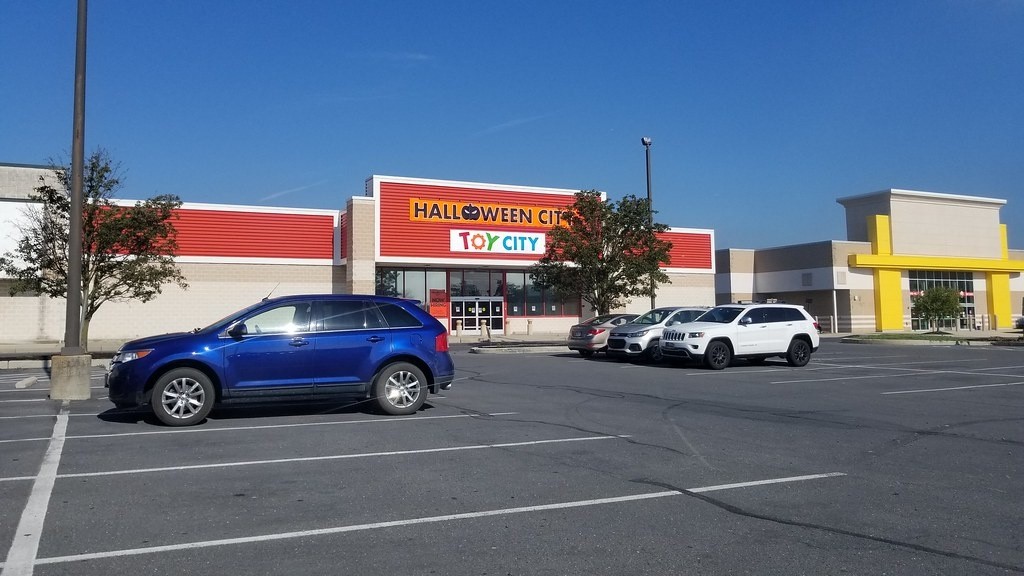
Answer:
[659,300,821,367]
[101,294,454,427]
[607,306,724,364]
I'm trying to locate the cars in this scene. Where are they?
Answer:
[568,313,658,357]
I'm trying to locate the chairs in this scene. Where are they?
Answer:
[292,308,310,331]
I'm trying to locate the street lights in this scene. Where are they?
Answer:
[642,136,657,320]
